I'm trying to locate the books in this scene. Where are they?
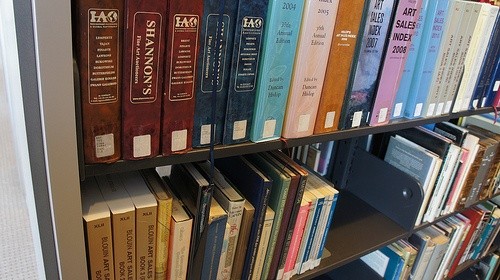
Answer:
[78,0,500,164]
[81,120,500,280]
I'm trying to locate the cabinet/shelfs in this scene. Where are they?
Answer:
[1,0,500,280]
